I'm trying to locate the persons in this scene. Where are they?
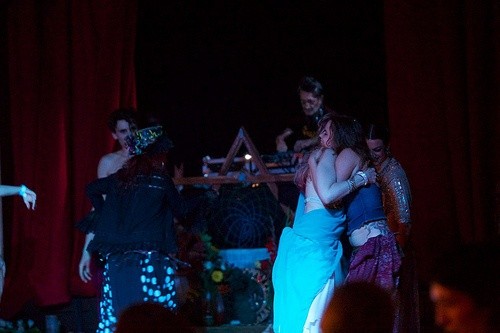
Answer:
[275,75,419,333]
[427,238,500,333]
[0,184,38,210]
[78,106,200,333]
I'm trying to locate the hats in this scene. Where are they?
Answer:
[124,127,172,157]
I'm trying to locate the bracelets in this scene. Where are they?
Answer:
[347,176,357,192]
[357,170,369,186]
[19,184,28,197]
